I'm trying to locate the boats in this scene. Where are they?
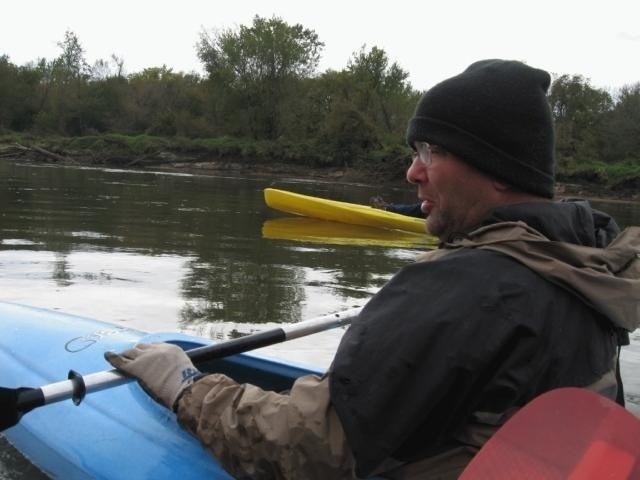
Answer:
[264,188,427,234]
[1,302,328,475]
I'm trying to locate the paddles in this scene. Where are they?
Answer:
[1,307,365,435]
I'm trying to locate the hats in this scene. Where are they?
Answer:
[406,59,554,199]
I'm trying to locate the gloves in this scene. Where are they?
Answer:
[103,341,203,410]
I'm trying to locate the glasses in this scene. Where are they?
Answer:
[410,142,447,166]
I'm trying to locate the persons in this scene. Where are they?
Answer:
[104,60,640,480]
